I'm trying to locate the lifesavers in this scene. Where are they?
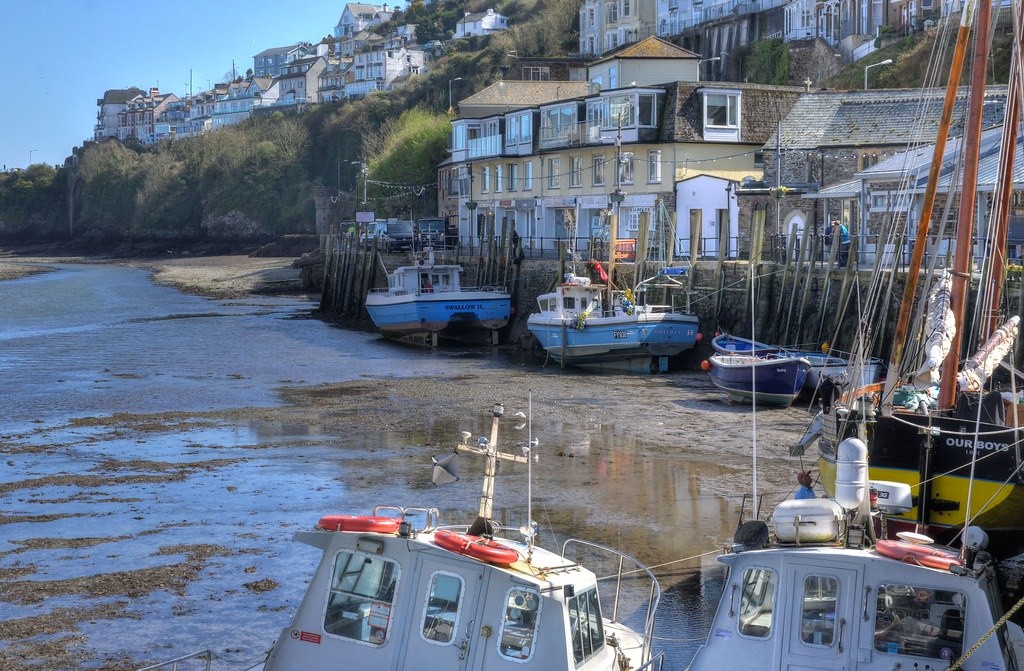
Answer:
[320,515,403,534]
[433,530,519,564]
[875,538,962,570]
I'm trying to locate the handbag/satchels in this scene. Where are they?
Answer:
[820,371,849,414]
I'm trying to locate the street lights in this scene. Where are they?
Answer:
[864,59,893,90]
[448,77,462,107]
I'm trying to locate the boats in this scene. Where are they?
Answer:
[133,388,666,671]
[701,349,812,408]
[367,264,512,349]
[711,331,884,396]
[685,437,1024,671]
[527,272,700,374]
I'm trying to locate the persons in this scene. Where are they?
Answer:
[794,470,828,499]
[513,231,524,259]
[832,220,851,267]
[424,279,434,293]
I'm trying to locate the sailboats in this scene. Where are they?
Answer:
[787,0,1023,626]
